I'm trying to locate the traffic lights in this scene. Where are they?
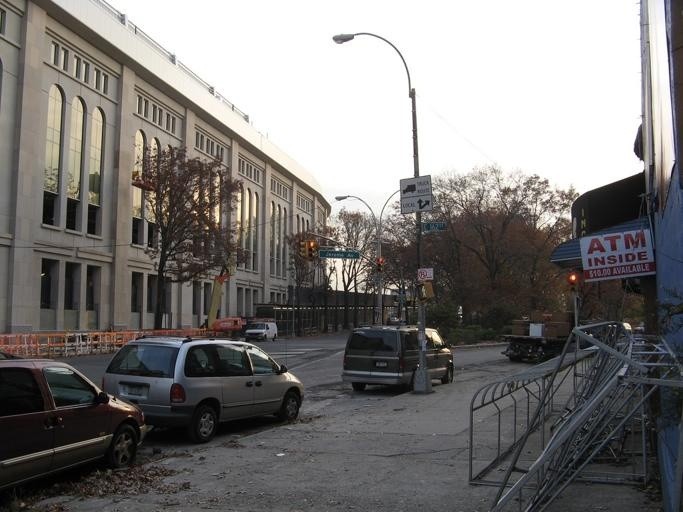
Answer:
[568,272,579,283]
[298,239,319,262]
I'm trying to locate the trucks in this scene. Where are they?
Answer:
[500,319,609,365]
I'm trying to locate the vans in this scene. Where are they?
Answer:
[102,333,305,443]
[0,355,149,495]
[243,322,277,343]
[340,324,455,393]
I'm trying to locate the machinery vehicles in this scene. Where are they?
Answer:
[203,258,242,331]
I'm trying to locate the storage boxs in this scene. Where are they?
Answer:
[512,312,570,337]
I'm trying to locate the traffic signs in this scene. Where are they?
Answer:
[400,194,432,214]
[319,251,361,259]
[420,221,447,233]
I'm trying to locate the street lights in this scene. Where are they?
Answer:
[332,32,430,394]
[334,193,381,324]
